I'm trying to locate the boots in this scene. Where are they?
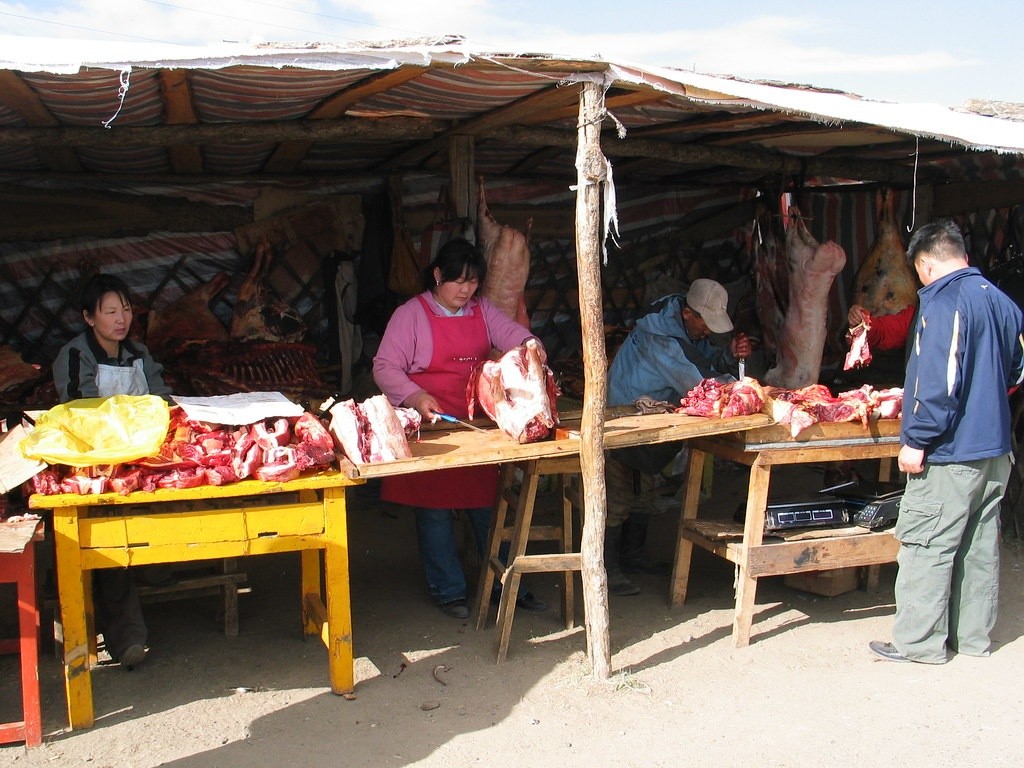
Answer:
[619,512,672,575]
[604,524,641,596]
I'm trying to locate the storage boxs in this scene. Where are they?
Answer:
[785,565,860,596]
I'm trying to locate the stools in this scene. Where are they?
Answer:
[469,454,584,664]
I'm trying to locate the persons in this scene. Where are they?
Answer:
[51,273,178,669]
[373,240,547,617]
[822,220,1024,664]
[604,279,753,595]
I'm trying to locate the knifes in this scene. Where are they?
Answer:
[739,356,745,380]
[434,411,489,434]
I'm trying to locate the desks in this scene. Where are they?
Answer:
[668,433,908,646]
[30,468,368,731]
[1,517,46,748]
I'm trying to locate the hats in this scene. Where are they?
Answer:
[686,278,734,334]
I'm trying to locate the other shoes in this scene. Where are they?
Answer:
[869,640,916,663]
[515,591,547,611]
[438,599,470,619]
[121,643,146,674]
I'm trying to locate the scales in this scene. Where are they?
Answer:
[820,481,907,530]
[733,493,851,532]
